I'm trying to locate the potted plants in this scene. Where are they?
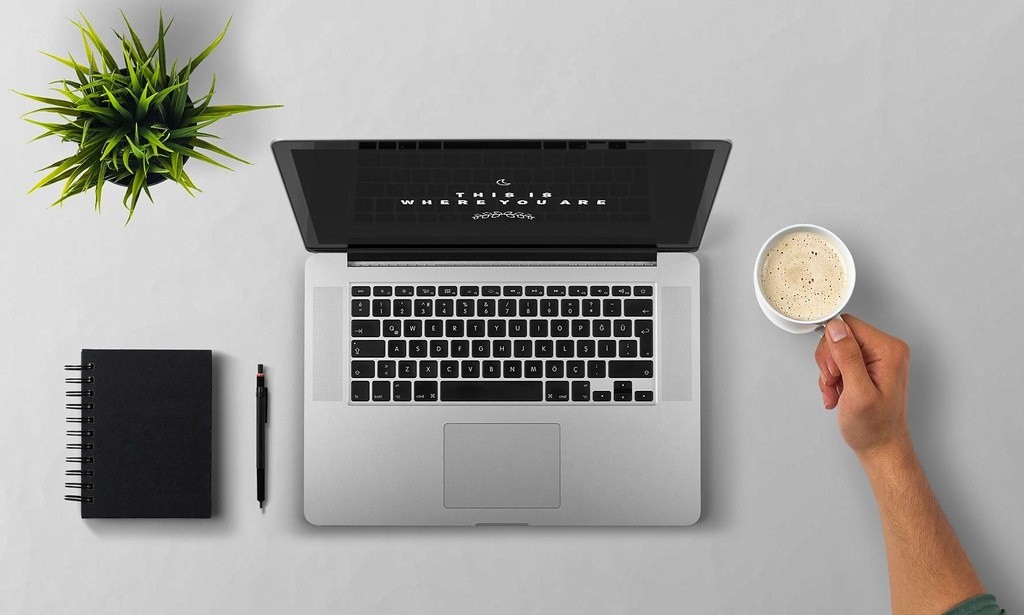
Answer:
[9,6,286,228]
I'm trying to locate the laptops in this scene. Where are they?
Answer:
[269,137,732,528]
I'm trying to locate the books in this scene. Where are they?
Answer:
[63,349,212,519]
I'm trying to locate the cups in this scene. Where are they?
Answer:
[752,223,857,335]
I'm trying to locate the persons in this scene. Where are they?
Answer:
[815,314,1006,615]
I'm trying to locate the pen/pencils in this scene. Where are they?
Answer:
[255,363,268,508]
